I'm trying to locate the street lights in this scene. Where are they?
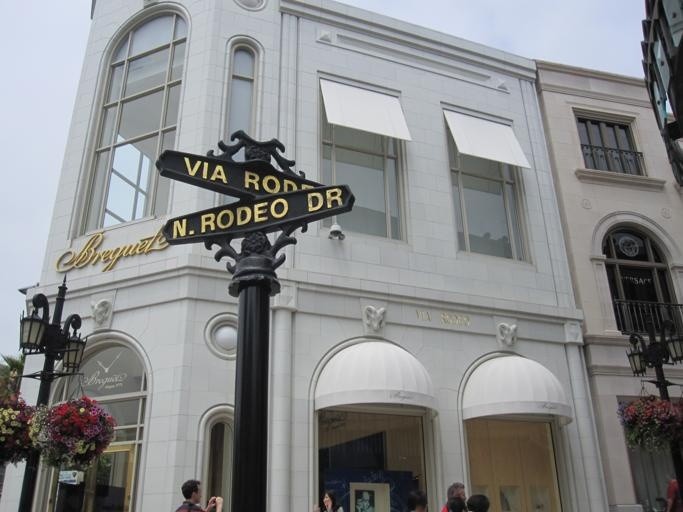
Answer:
[624,315,682,510]
[17,273,87,512]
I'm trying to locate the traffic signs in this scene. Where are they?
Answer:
[155,150,325,199]
[161,184,354,245]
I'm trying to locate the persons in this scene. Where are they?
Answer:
[402,489,427,512]
[175,478,223,512]
[355,491,372,511]
[446,495,465,512]
[321,490,343,511]
[663,474,682,512]
[465,493,489,512]
[653,496,668,512]
[439,481,467,512]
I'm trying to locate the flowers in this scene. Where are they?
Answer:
[0,390,37,464]
[27,396,117,470]
[616,396,682,458]
